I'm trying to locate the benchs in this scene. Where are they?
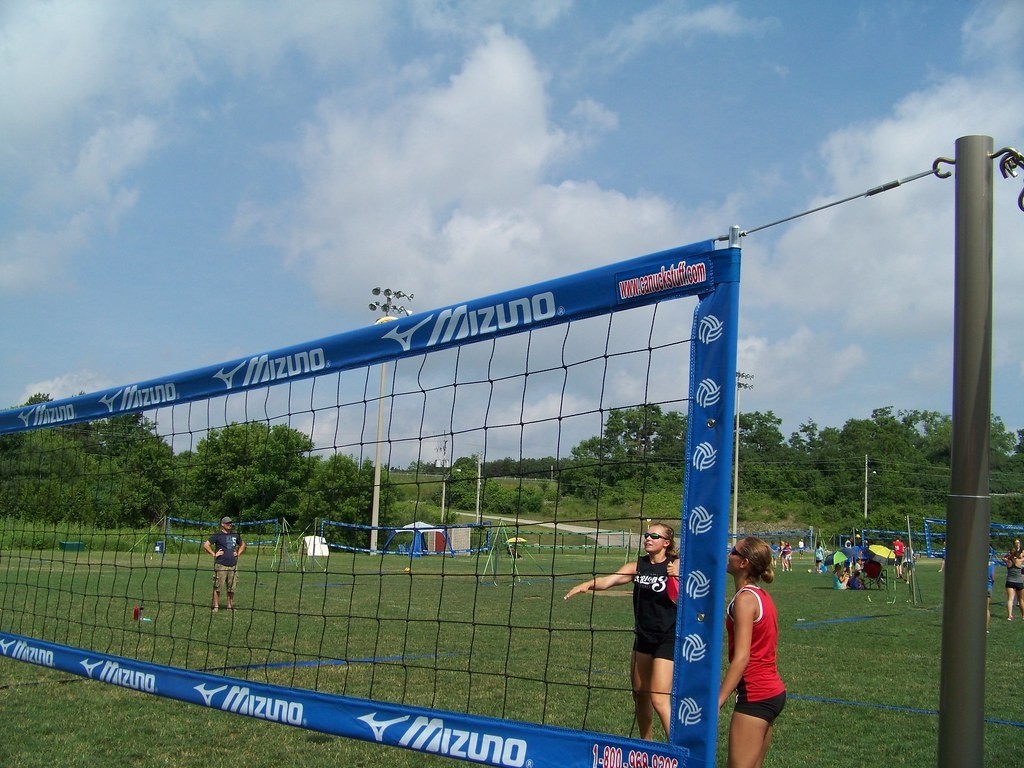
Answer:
[60,540,85,552]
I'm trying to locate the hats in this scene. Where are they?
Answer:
[507,544,513,548]
[222,516,234,524]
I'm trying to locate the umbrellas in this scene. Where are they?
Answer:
[505,537,527,550]
[824,548,856,576]
[868,544,895,564]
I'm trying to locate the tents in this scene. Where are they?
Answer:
[380,521,457,559]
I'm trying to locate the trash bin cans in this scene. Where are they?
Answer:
[155,541,164,554]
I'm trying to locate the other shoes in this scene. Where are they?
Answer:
[937,569,942,572]
[986,630,990,633]
[1007,616,1013,621]
[789,569,792,571]
[212,609,218,613]
[900,574,903,579]
[227,607,237,612]
[1022,616,1024,620]
[785,568,789,571]
[1016,601,1019,607]
[906,581,909,584]
[782,569,784,571]
[896,576,900,579]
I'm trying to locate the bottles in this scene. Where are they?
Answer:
[140,618,152,622]
[133,606,138,620]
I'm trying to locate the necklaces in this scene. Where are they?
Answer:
[650,557,666,564]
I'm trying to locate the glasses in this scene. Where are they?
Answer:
[643,532,668,540]
[729,546,747,559]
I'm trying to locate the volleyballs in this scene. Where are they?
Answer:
[856,534,861,538]
[807,569,812,573]
[405,567,410,573]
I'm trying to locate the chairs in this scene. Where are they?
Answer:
[862,569,888,591]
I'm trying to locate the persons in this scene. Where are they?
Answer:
[202,517,244,612]
[769,539,804,571]
[506,544,523,559]
[814,535,917,592]
[718,536,786,768]
[985,538,1024,634]
[563,523,680,741]
[937,540,946,573]
